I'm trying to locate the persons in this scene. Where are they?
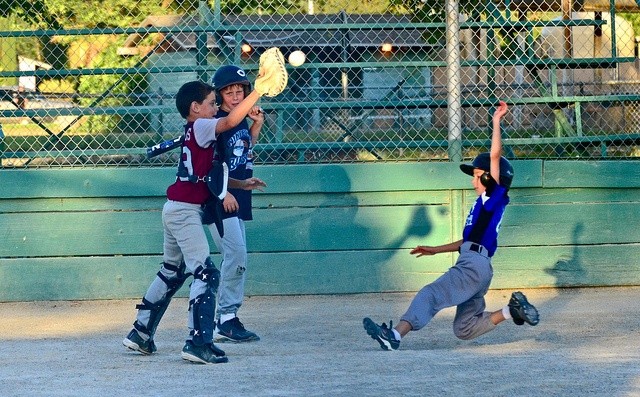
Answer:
[123,45,288,363]
[362,101,540,352]
[205,66,267,342]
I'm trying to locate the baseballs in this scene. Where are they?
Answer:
[288,50,306,67]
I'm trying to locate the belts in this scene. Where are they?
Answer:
[459,244,481,253]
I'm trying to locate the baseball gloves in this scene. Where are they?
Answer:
[253,46,288,98]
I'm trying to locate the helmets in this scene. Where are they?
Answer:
[461,153,514,188]
[210,65,251,107]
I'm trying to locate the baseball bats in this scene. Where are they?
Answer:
[146,108,264,160]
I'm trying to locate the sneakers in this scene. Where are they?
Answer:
[182,340,228,364]
[122,329,158,354]
[509,292,539,326]
[363,317,400,351]
[215,318,260,343]
